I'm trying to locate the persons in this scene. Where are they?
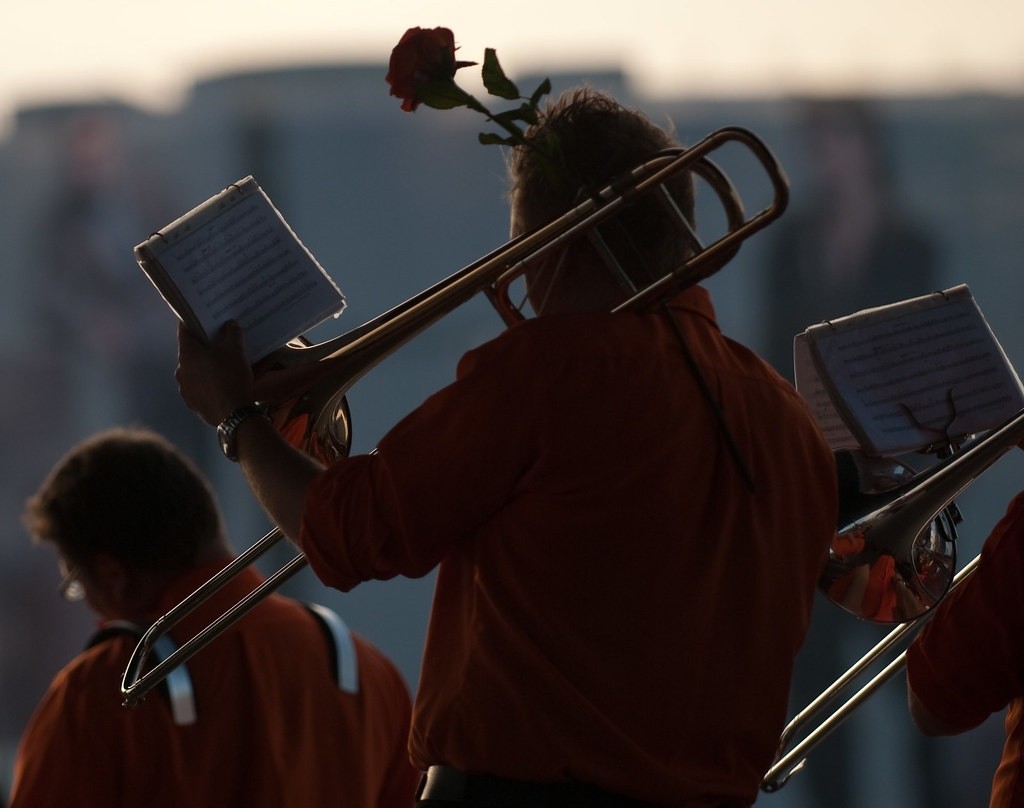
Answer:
[7,429,424,808]
[906,491,1024,808]
[173,90,838,808]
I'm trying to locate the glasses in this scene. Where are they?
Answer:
[55,563,95,602]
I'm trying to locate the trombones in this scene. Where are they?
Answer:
[758,405,1024,795]
[120,124,790,711]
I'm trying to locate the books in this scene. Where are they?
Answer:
[134,174,348,369]
[795,283,1024,458]
